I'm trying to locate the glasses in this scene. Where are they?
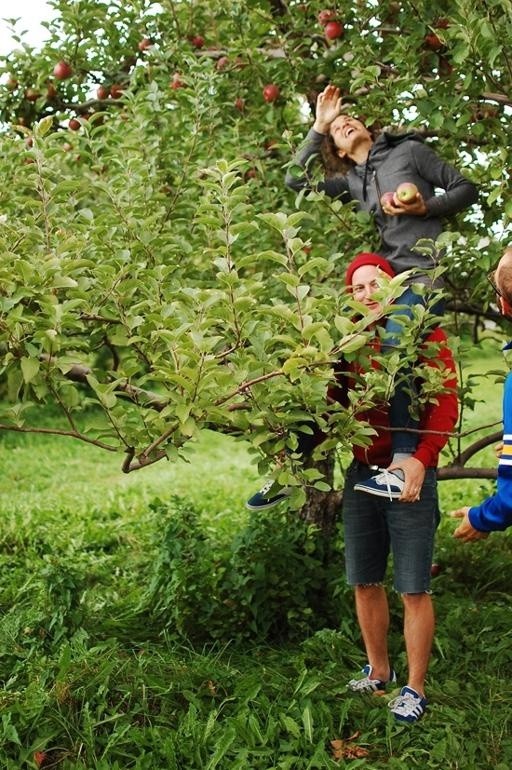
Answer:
[487,269,505,298]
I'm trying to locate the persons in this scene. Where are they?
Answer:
[447,242,512,543]
[280,250,462,722]
[245,82,480,514]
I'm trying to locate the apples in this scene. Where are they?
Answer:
[7,4,498,212]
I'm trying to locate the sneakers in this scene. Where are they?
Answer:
[346,664,396,695]
[245,479,287,510]
[388,686,427,722]
[353,471,420,500]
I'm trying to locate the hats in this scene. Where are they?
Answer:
[346,253,395,293]
